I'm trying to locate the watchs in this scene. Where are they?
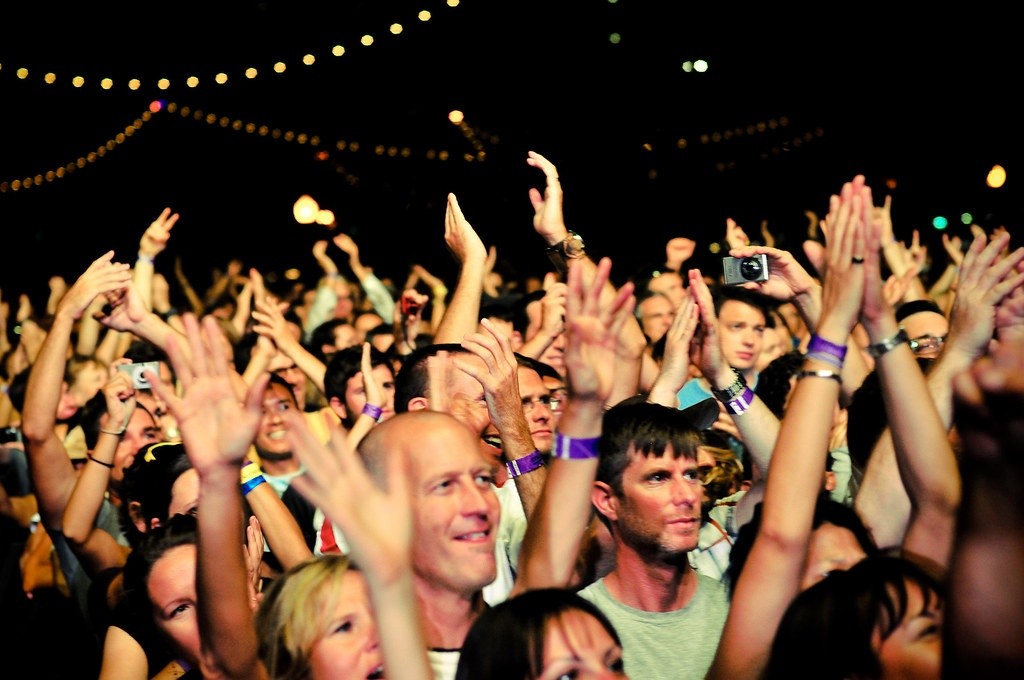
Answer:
[710,368,747,402]
[546,229,586,269]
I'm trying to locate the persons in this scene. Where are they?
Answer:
[1,149,1023,680]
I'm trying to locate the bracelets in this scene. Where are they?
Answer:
[360,402,382,419]
[237,461,263,484]
[159,308,177,322]
[550,431,601,460]
[505,449,546,479]
[867,328,912,359]
[807,334,848,362]
[240,475,266,496]
[88,453,114,470]
[137,256,155,264]
[797,370,843,389]
[723,388,754,416]
[805,351,844,368]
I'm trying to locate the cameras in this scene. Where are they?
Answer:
[117,362,160,389]
[400,296,421,316]
[722,254,770,286]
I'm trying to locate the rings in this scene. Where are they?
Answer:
[852,257,864,266]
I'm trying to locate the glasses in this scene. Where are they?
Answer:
[519,395,562,416]
[910,335,949,355]
